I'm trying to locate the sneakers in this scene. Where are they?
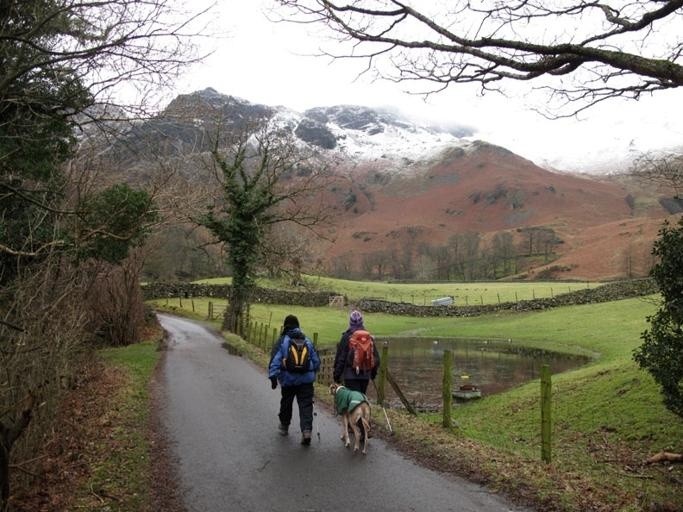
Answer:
[278,423,289,437]
[300,428,312,444]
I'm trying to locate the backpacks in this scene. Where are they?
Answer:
[346,329,374,376]
[287,337,310,374]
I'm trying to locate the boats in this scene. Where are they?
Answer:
[451,384,481,400]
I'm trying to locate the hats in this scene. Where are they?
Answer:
[283,315,299,328]
[349,310,363,326]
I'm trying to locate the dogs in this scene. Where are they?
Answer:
[328,383,370,455]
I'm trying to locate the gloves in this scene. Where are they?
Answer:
[271,378,278,391]
[370,372,378,380]
[333,371,342,383]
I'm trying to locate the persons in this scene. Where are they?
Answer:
[269,315,321,444]
[333,310,380,441]
